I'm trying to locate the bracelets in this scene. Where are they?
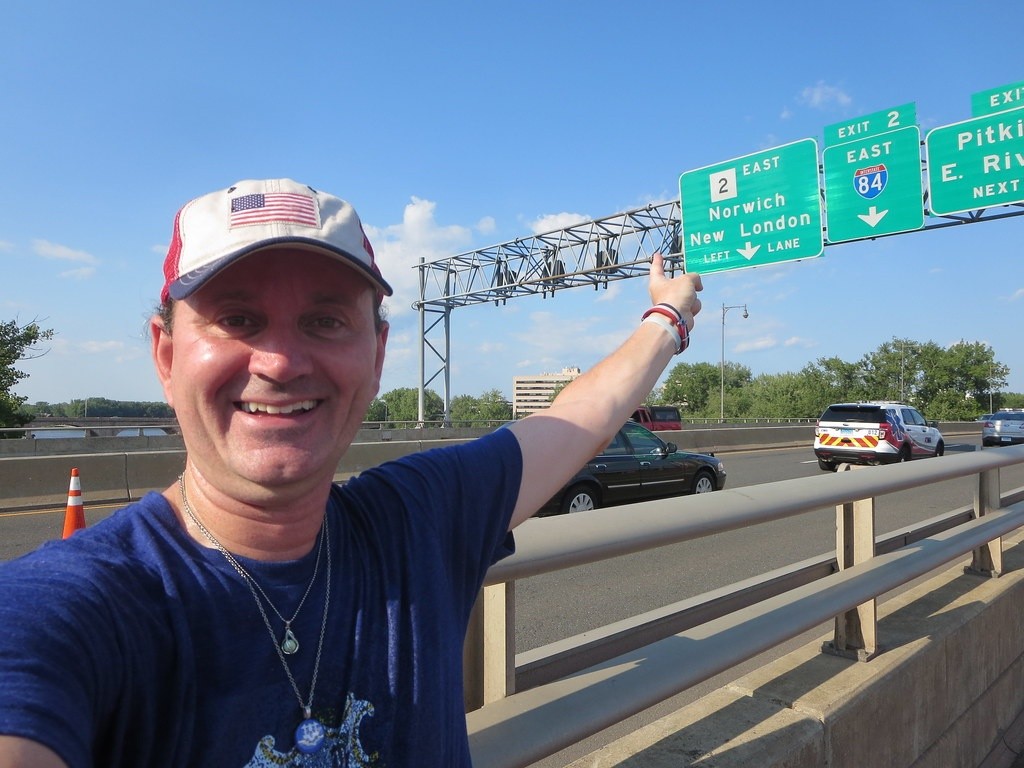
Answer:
[640,303,689,354]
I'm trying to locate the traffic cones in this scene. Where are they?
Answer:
[60,467,87,539]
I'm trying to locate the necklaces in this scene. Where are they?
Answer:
[178,471,331,754]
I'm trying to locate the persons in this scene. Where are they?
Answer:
[0,178,703,768]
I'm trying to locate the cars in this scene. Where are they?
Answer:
[982,411,1024,447]
[532,420,726,519]
[975,414,994,421]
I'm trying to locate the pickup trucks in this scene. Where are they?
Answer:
[631,406,681,431]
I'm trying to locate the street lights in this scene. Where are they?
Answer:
[990,364,1007,415]
[721,301,749,419]
[901,342,922,404]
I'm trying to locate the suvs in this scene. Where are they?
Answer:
[813,401,945,469]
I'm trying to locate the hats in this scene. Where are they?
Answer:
[160,178,393,311]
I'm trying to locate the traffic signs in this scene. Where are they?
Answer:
[822,100,926,245]
[678,136,825,277]
